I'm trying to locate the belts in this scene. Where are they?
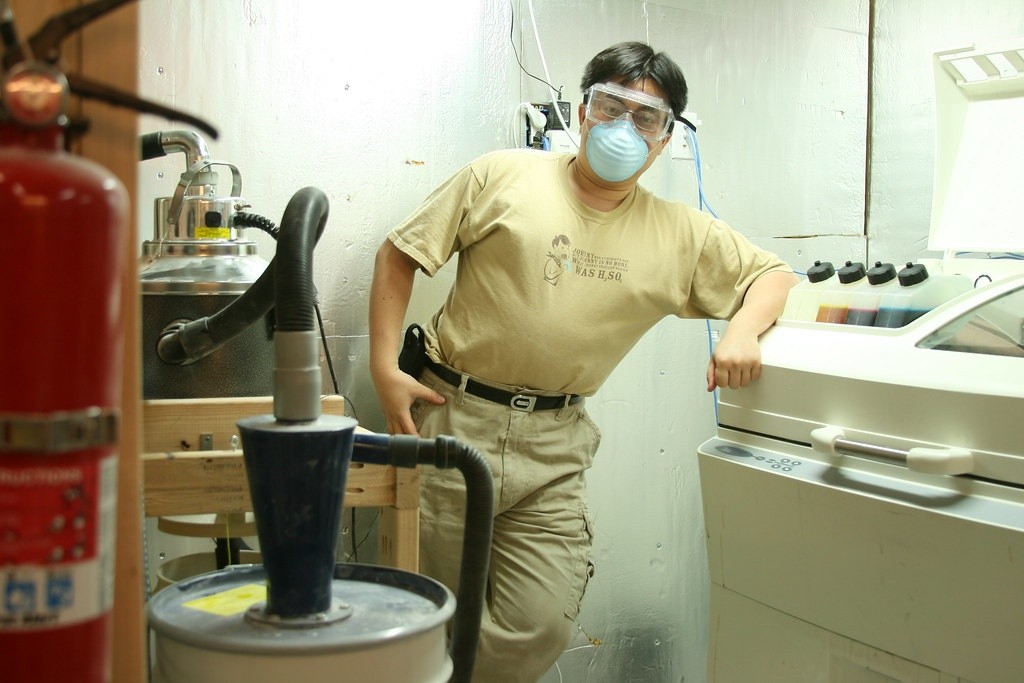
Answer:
[423,355,585,412]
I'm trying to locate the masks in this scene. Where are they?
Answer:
[584,120,663,182]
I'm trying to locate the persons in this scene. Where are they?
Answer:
[369,42,801,683]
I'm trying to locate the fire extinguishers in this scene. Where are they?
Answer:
[0,0,219,683]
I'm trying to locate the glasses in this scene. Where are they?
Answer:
[584,81,676,143]
